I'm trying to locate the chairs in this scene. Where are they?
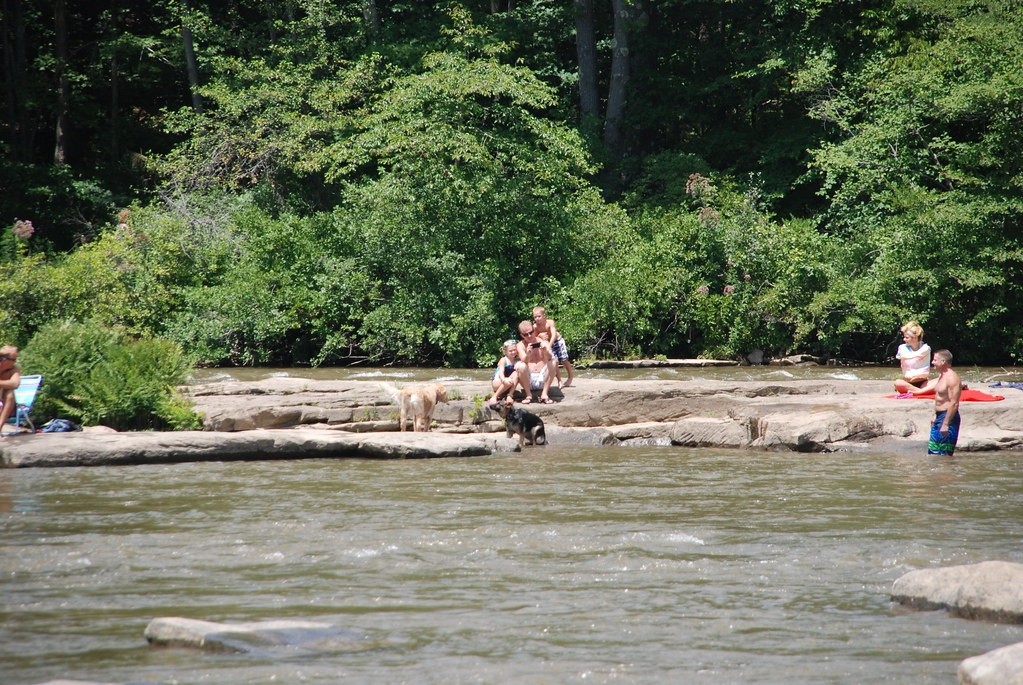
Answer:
[8,374,43,435]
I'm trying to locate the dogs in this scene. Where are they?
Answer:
[489,396,548,447]
[399,384,450,432]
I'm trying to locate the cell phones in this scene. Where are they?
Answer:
[531,342,541,348]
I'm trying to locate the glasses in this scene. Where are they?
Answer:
[504,340,516,345]
[523,329,534,337]
[6,357,16,362]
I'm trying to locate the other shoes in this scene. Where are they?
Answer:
[522,396,535,404]
[540,395,557,404]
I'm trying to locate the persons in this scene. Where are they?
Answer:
[533,306,573,390]
[928,349,961,457]
[893,321,939,395]
[514,321,557,404]
[489,340,518,404]
[0,346,20,430]
